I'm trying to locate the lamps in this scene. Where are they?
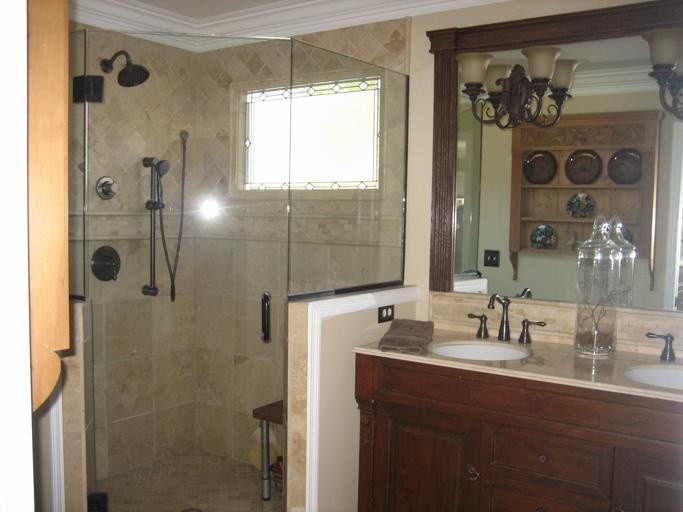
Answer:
[639,23,682,114]
[451,44,561,124]
[482,59,582,130]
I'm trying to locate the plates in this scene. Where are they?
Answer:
[566,192,595,219]
[523,151,557,184]
[564,150,602,185]
[529,224,557,250]
[607,148,642,184]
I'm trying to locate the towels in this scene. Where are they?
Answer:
[375,317,435,357]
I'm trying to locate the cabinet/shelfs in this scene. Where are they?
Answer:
[615,446,683,512]
[476,421,616,510]
[369,403,483,510]
[505,109,665,292]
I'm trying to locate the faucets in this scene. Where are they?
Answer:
[516,287,533,299]
[488,294,510,341]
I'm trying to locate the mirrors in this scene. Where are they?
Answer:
[422,0,683,314]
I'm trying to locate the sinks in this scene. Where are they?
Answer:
[624,364,682,391]
[427,340,530,361]
